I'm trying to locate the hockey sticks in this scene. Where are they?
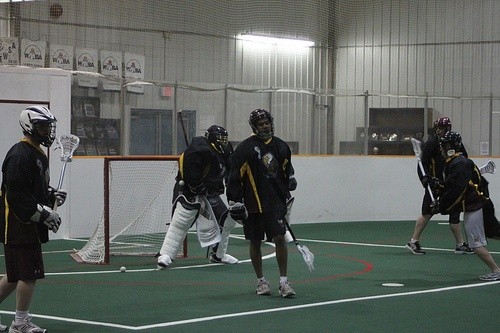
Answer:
[178,109,189,148]
[53,134,80,213]
[281,215,315,272]
[479,161,495,174]
[411,138,435,202]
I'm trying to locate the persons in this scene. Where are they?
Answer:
[0,106,67,333]
[157,125,238,270]
[226,109,297,297]
[406,117,475,254]
[422,131,500,280]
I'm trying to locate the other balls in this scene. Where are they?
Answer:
[120,266,126,272]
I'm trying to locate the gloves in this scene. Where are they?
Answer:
[228,200,248,224]
[52,189,67,206]
[428,201,442,214]
[43,213,61,233]
[422,175,440,188]
[288,176,297,191]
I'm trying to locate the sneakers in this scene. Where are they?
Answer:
[9,316,47,333]
[454,241,475,254]
[405,239,426,254]
[0,325,8,330]
[478,270,500,281]
[157,255,172,269]
[256,280,272,295]
[279,282,296,298]
[209,253,238,264]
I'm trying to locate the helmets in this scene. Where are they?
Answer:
[248,108,275,139]
[19,106,57,147]
[204,124,228,158]
[434,117,452,136]
[439,131,461,156]
